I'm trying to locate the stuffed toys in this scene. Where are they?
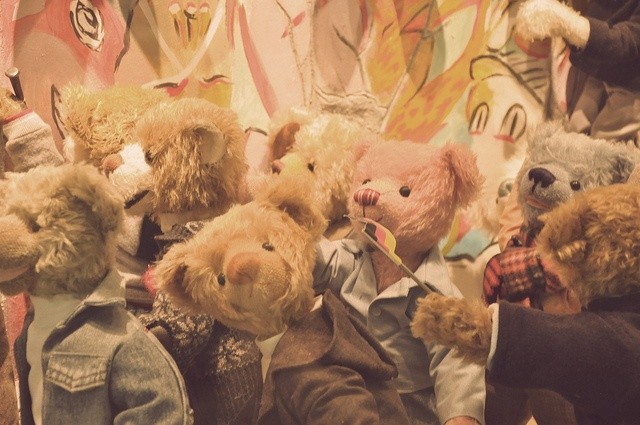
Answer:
[152,199,409,425]
[309,139,486,425]
[497,121,640,319]
[411,184,640,425]
[0,84,171,177]
[103,97,261,425]
[0,164,193,425]
[515,0,639,145]
[265,109,378,241]
